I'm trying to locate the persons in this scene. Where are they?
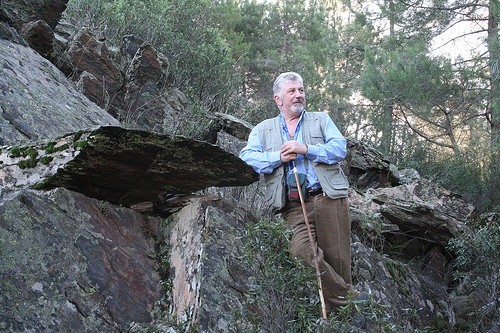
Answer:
[239,72,361,317]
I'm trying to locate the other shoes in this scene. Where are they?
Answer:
[345,291,368,326]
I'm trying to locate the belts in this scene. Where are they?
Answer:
[308,188,322,196]
